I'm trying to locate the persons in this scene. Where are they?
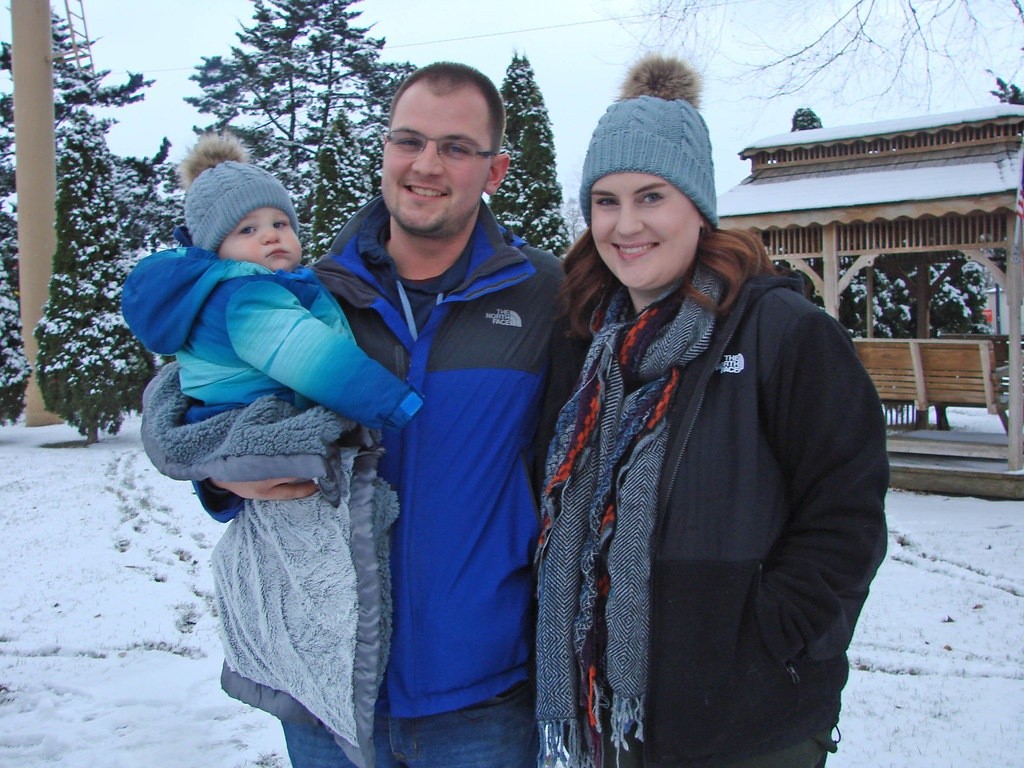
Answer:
[189,62,572,768]
[525,56,889,767]
[114,128,426,499]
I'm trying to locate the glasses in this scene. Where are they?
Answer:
[384,127,495,168]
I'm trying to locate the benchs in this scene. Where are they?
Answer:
[849,333,1024,438]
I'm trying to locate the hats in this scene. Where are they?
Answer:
[579,56,721,228]
[179,131,300,250]
